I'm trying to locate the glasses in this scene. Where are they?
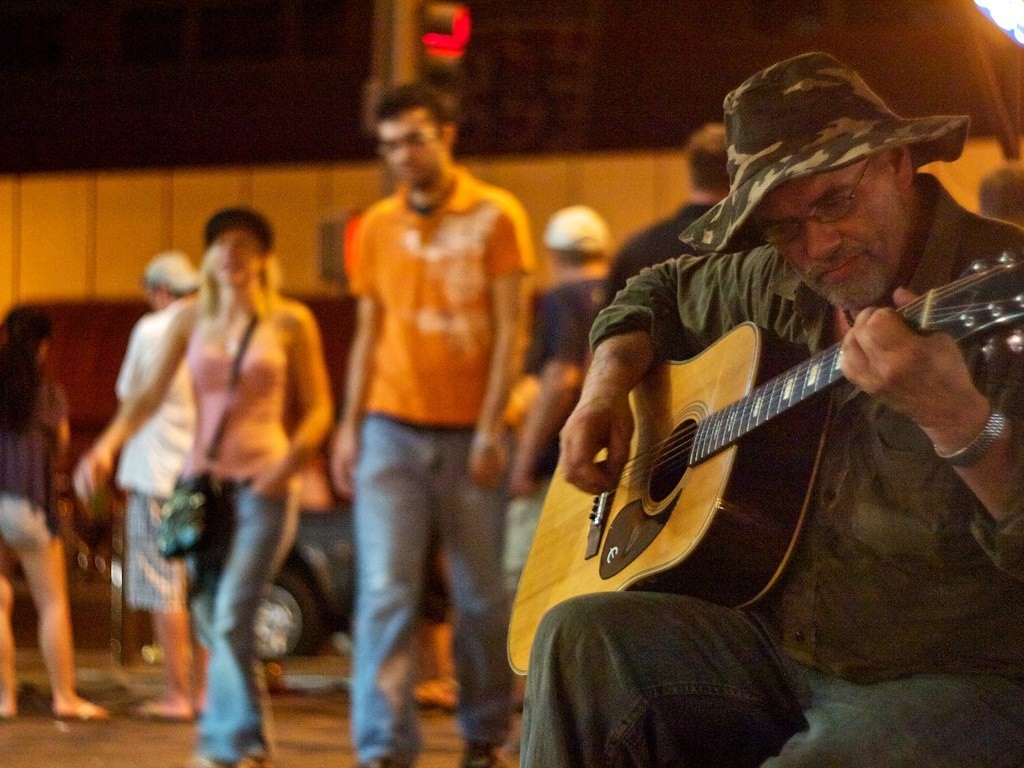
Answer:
[374,125,444,156]
[748,156,874,244]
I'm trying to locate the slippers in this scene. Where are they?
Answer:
[126,699,193,723]
[54,702,107,723]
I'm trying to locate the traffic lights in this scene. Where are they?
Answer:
[380,0,472,125]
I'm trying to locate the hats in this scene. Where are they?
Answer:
[545,204,608,255]
[144,250,201,294]
[678,52,969,254]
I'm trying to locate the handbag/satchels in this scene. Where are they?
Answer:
[157,474,220,557]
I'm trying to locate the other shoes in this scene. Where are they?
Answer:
[365,757,410,768]
[199,754,267,768]
[462,743,496,768]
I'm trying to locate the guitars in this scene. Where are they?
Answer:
[505,251,1024,678]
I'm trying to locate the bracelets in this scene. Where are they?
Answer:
[932,402,1008,467]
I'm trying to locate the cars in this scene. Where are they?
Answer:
[245,495,451,667]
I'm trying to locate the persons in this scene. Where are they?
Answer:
[331,81,535,768]
[71,121,1024,768]
[517,50,1024,768]
[0,298,112,719]
